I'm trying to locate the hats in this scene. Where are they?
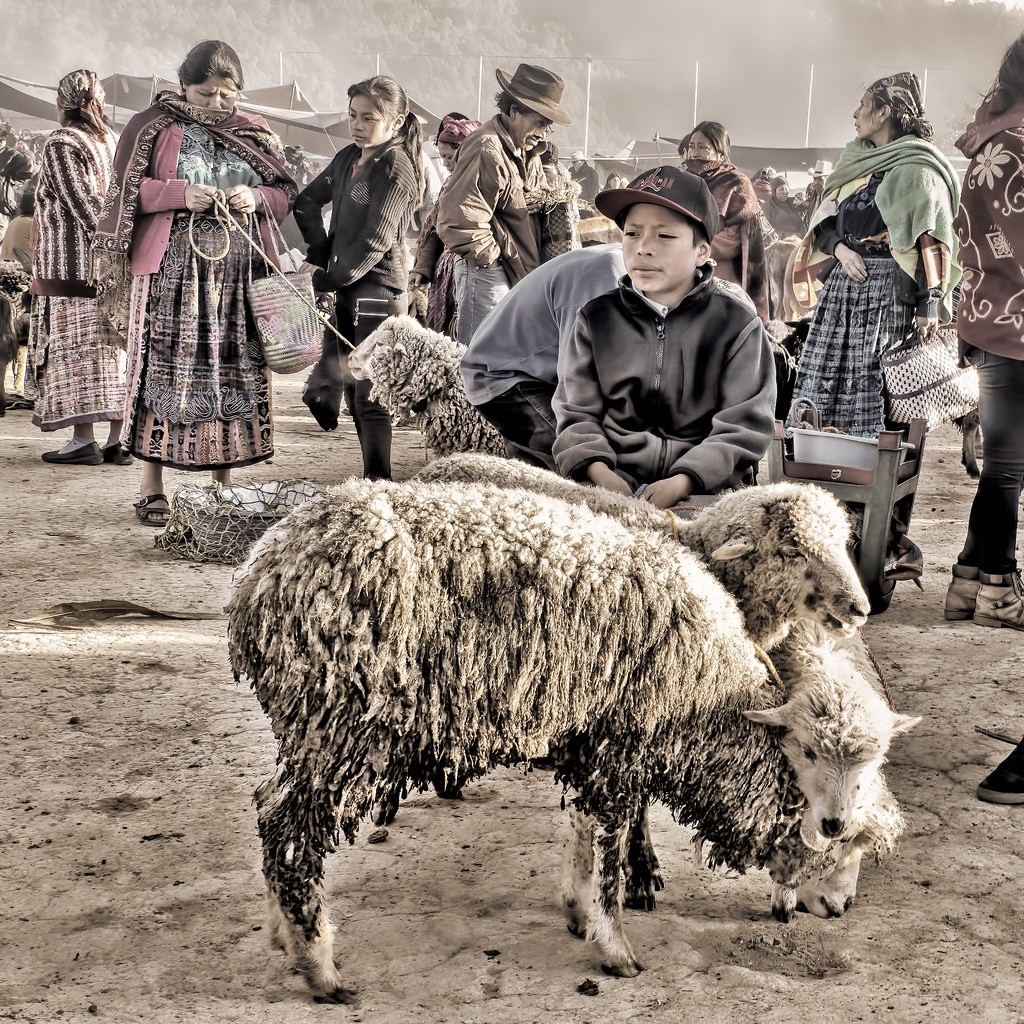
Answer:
[496,64,574,127]
[807,160,833,177]
[569,152,588,162]
[595,165,719,245]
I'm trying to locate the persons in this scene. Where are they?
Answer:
[782,72,963,443]
[976,736,1024,805]
[436,61,572,347]
[678,132,779,252]
[945,31,1024,633]
[31,68,135,466]
[1,191,34,274]
[567,151,601,204]
[677,120,771,324]
[460,240,628,474]
[293,75,424,483]
[92,40,299,529]
[434,112,471,146]
[752,160,833,239]
[0,127,34,247]
[551,164,777,513]
[540,141,571,186]
[409,115,483,334]
[602,172,626,191]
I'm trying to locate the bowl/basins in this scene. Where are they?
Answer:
[788,427,916,470]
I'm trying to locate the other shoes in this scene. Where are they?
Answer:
[944,561,1024,632]
[978,733,1024,804]
[101,442,134,465]
[41,441,104,466]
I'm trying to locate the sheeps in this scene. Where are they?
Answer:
[373,442,872,908]
[343,313,514,455]
[224,477,928,1004]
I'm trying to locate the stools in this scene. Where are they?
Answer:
[764,406,931,621]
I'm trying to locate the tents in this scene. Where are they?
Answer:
[0,71,448,163]
[594,136,844,177]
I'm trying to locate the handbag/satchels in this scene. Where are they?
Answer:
[246,189,325,375]
[882,326,980,435]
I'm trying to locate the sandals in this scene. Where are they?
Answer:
[133,494,171,527]
